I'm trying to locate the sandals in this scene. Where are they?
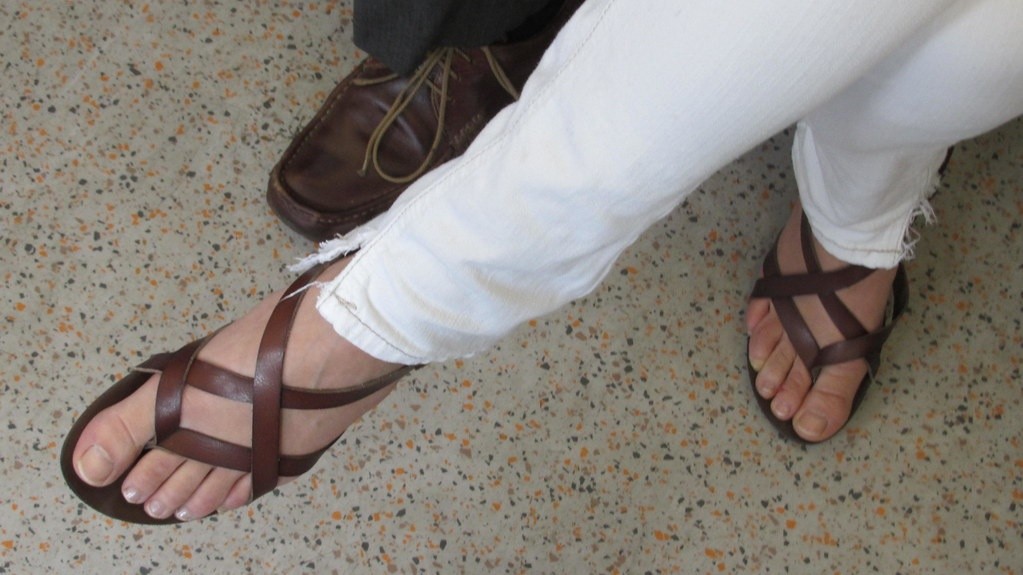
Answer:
[747,145,955,443]
[62,247,428,527]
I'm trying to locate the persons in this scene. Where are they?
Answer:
[60,0,1023,526]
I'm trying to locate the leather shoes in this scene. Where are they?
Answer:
[267,31,557,241]
